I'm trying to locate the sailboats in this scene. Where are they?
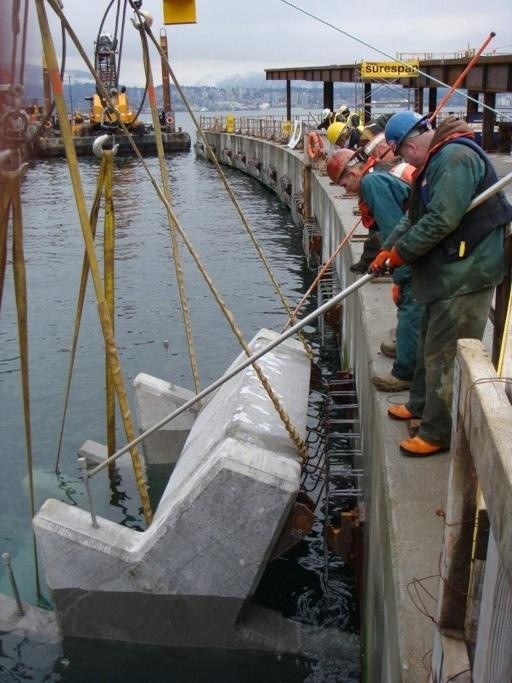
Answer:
[35,0,195,159]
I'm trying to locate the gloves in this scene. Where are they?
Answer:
[369,246,405,272]
[392,285,400,306]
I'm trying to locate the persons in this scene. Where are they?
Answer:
[315,104,416,391]
[370,110,512,456]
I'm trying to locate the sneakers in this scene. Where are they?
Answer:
[380,342,397,358]
[400,435,441,456]
[388,404,414,420]
[372,373,411,392]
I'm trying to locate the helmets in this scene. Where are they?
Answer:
[326,146,362,184]
[385,111,433,156]
[322,105,348,145]
